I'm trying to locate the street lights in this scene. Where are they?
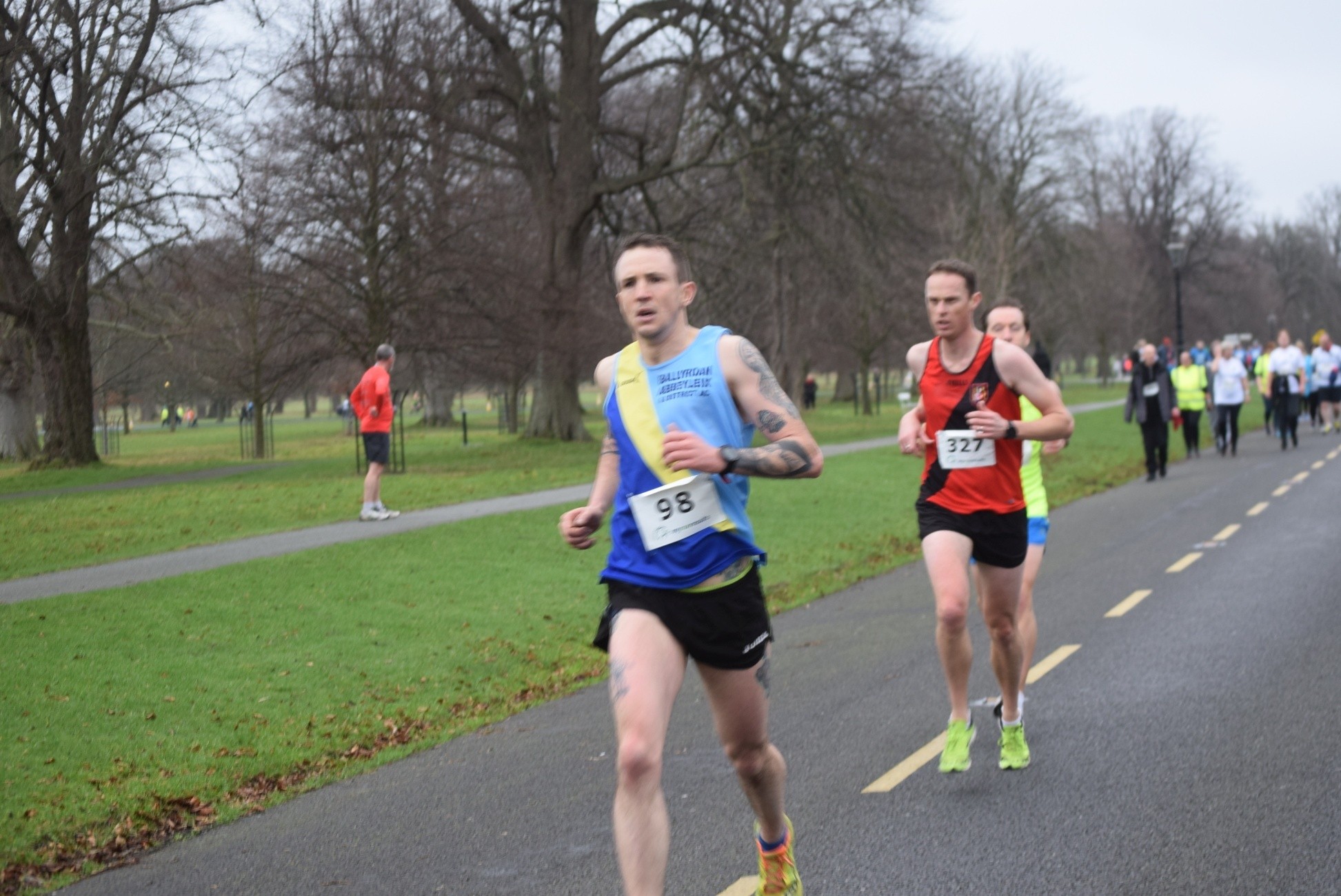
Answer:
[1167,228,1189,365]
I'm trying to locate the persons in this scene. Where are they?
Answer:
[804,373,817,410]
[899,266,1341,773]
[350,343,401,523]
[559,236,825,895]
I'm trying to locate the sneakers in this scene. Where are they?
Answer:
[938,708,978,774]
[358,504,400,521]
[753,812,805,896]
[997,716,1031,770]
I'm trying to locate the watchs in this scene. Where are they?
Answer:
[1005,419,1016,440]
[715,445,740,477]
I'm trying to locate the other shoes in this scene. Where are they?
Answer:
[1146,466,1167,481]
